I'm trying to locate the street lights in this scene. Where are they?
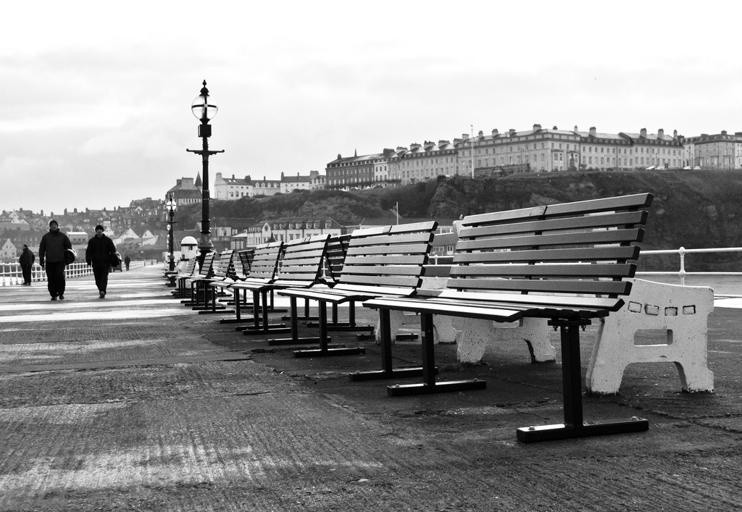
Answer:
[164,191,179,287]
[186,76,224,273]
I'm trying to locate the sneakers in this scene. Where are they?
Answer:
[99,290,105,298]
[51,296,64,300]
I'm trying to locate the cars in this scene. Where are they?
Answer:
[110,251,124,274]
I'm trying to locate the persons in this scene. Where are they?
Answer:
[125,256,130,270]
[19,244,35,285]
[85,225,116,298]
[39,219,72,300]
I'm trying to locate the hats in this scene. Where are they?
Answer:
[95,225,104,232]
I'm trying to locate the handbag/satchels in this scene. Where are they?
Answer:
[112,251,122,266]
[65,249,76,265]
